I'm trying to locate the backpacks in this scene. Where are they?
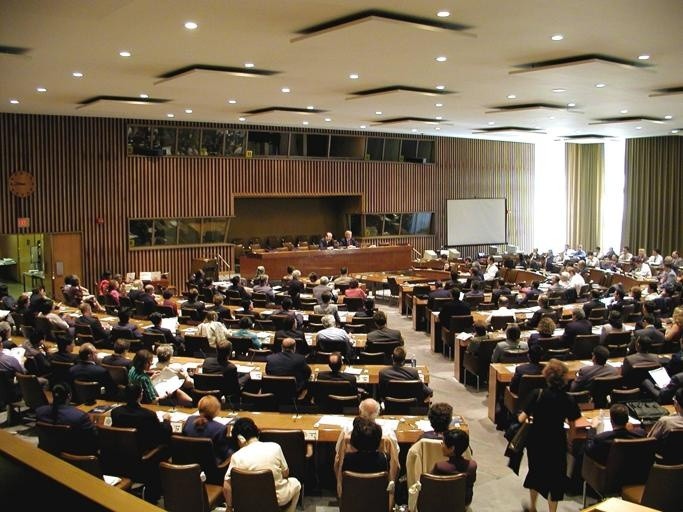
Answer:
[625,398,670,423]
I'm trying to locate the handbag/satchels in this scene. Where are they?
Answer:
[509,416,535,456]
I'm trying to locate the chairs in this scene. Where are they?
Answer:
[0,259,683,512]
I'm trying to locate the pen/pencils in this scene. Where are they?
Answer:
[408,423,415,429]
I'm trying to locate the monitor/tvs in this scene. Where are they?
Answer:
[489,246,497,255]
[508,245,518,253]
[423,250,437,262]
[449,248,460,261]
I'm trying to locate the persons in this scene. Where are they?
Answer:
[127,125,247,158]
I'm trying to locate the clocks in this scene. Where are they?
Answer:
[7,171,37,197]
